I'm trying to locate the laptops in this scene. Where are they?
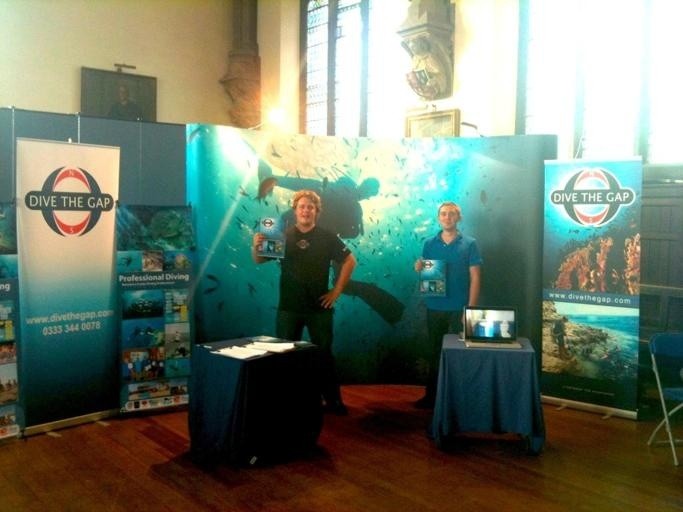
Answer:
[462,306,524,349]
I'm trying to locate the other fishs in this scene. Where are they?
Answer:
[230,136,498,318]
[216,300,225,312]
[207,274,221,285]
[204,287,219,294]
[189,127,201,142]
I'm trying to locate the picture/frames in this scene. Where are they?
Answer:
[405,109,460,136]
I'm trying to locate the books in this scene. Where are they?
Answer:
[416,258,447,298]
[256,215,289,258]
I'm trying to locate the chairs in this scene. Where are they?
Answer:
[644,330,683,466]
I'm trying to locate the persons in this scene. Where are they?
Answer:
[253,191,356,417]
[174,331,183,339]
[412,202,484,409]
[143,253,163,271]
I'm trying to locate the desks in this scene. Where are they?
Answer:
[187,334,322,467]
[435,331,537,454]
